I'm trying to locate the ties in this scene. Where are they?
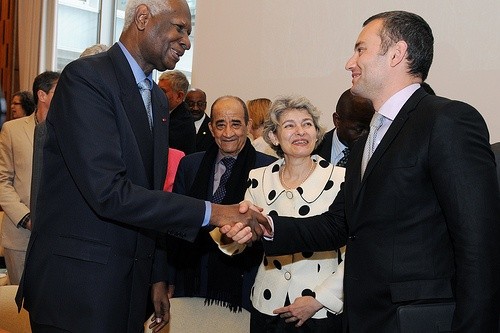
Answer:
[368,115,384,157]
[336,148,350,168]
[213,157,236,204]
[137,78,153,131]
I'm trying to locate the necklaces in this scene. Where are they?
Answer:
[282,159,314,190]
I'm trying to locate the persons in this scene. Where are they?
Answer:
[15,1,274,333]
[246,98,282,159]
[185,89,217,150]
[311,87,374,169]
[158,69,195,150]
[220,12,500,333]
[242,91,348,333]
[0,41,112,285]
[172,95,279,313]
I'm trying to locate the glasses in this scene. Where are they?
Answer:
[12,102,21,105]
